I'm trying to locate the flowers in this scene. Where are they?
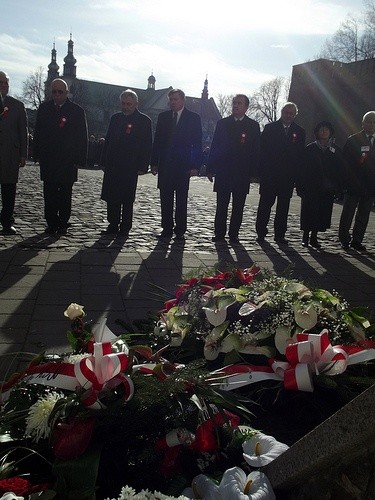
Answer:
[0,262,375,499]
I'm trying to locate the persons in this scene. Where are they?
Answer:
[295,121,345,247]
[86,133,106,168]
[151,89,203,239]
[99,89,152,234]
[32,79,88,233]
[253,102,306,238]
[0,72,28,234]
[336,111,375,251]
[206,95,260,242]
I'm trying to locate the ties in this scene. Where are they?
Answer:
[284,123,289,131]
[236,119,240,127]
[55,104,60,112]
[173,111,177,122]
[367,135,374,147]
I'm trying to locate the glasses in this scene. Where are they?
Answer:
[51,89,65,95]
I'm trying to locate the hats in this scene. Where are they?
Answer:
[314,121,333,138]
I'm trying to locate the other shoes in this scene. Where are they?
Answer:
[1,226,17,236]
[273,234,289,243]
[256,227,270,240]
[156,229,185,240]
[43,225,70,234]
[302,231,321,248]
[212,233,224,241]
[228,236,240,243]
[339,240,366,251]
[101,227,129,239]
